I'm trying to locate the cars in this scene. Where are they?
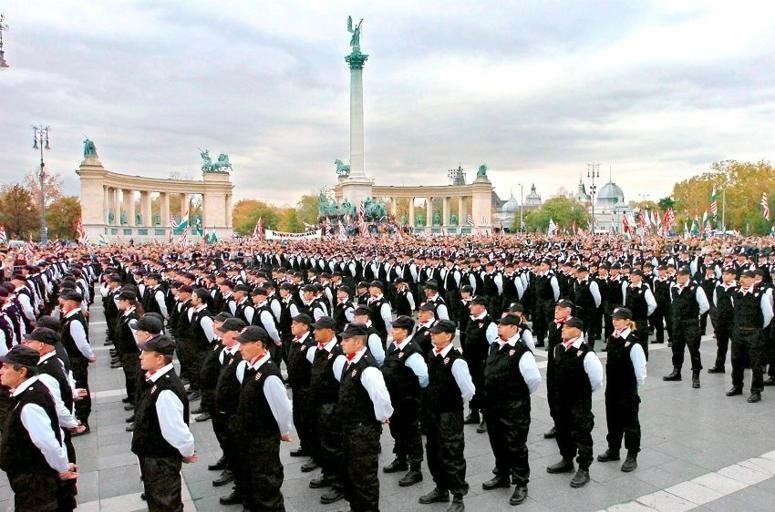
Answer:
[663,228,737,239]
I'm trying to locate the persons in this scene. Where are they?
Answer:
[0,239,97,512]
[621,231,775,402]
[94,236,647,512]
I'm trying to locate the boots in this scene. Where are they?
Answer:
[663,368,682,381]
[691,370,700,388]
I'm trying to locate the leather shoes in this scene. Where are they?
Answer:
[547,460,576,473]
[570,469,590,487]
[708,365,726,373]
[598,449,621,462]
[110,353,119,369]
[747,393,761,403]
[509,486,528,505]
[621,456,637,472]
[178,375,211,422]
[544,427,556,438]
[383,459,465,511]
[764,375,774,386]
[726,386,744,396]
[122,396,133,431]
[207,463,240,506]
[477,421,487,433]
[482,476,511,490]
[290,448,345,504]
[463,414,482,424]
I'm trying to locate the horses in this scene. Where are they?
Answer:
[334,159,350,175]
[215,154,233,171]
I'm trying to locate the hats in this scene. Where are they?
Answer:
[1,256,84,366]
[543,248,766,277]
[553,299,633,330]
[275,271,415,342]
[102,264,273,352]
[418,280,522,332]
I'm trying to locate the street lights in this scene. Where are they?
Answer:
[717,183,734,232]
[588,160,601,236]
[518,183,524,234]
[637,193,650,236]
[448,168,465,185]
[32,124,50,247]
[0,16,10,72]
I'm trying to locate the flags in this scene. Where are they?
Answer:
[541,182,719,241]
[69,218,92,248]
[760,192,771,221]
[167,206,493,241]
